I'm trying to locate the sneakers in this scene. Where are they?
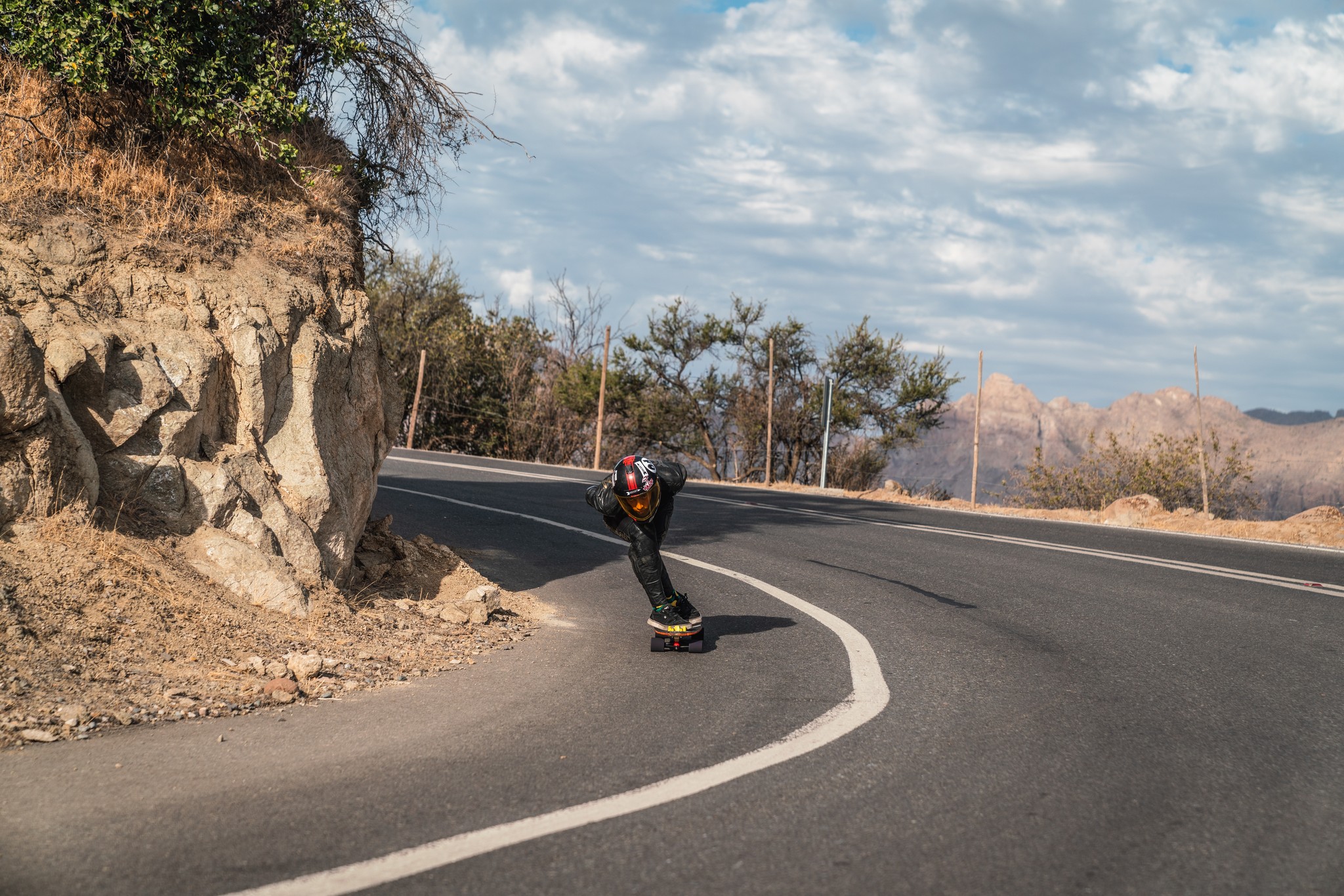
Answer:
[647,603,693,631]
[667,590,702,624]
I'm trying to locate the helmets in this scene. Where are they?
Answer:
[611,455,662,523]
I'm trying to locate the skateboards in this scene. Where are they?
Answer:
[649,600,705,653]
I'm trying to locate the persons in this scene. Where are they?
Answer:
[585,456,702,631]
